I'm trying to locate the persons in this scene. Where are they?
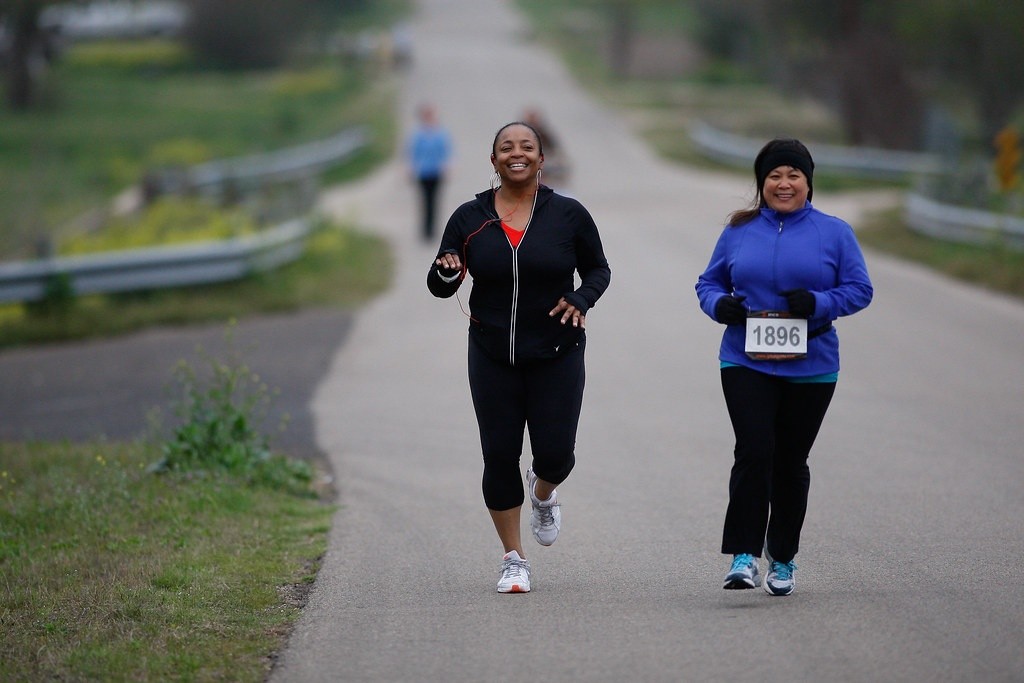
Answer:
[526,108,566,188]
[427,123,611,594]
[411,113,450,244]
[694,139,873,598]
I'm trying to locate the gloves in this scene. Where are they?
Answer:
[714,295,748,327]
[778,289,816,319]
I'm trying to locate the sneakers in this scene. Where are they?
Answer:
[762,539,799,596]
[526,465,562,546]
[723,554,762,588]
[496,549,531,593]
